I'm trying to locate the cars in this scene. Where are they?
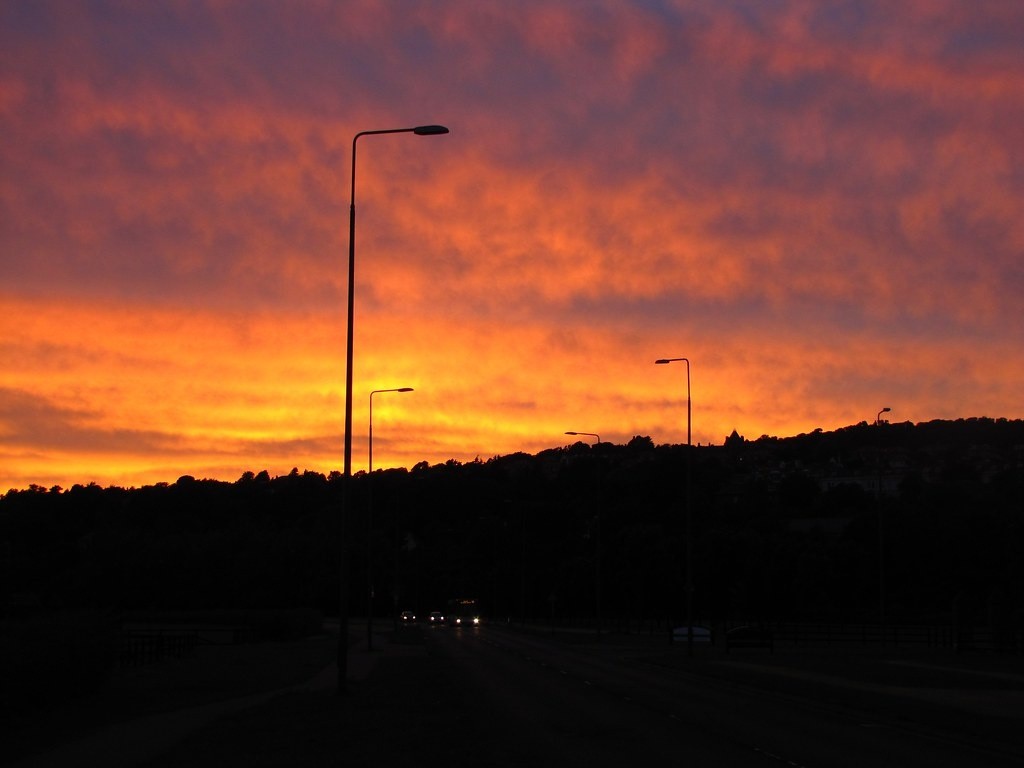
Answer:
[397,598,480,628]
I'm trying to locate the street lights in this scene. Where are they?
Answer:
[563,432,603,639]
[654,357,694,652]
[878,406,889,647]
[368,387,414,643]
[334,123,451,660]
